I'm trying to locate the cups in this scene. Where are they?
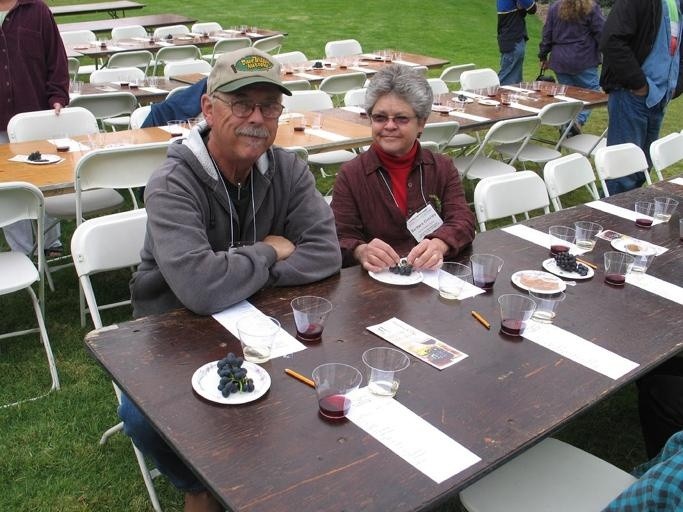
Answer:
[437,252,567,340]
[287,107,329,141]
[358,338,406,400]
[69,68,159,94]
[678,218,682,239]
[633,201,656,228]
[652,196,680,224]
[308,361,363,424]
[284,47,403,72]
[547,220,657,287]
[59,118,211,159]
[231,310,280,367]
[431,78,576,118]
[287,285,331,347]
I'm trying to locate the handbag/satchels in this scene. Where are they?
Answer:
[533,64,556,97]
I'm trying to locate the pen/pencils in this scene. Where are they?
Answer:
[576,258,597,270]
[284,368,318,388]
[472,311,490,328]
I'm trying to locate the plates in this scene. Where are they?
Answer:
[187,357,278,409]
[367,261,424,287]
[24,153,61,165]
[542,257,596,280]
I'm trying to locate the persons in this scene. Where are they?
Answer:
[635,356,683,460]
[0,0,70,258]
[330,64,477,272]
[598,0,683,197]
[497,0,537,86]
[537,0,606,138]
[116,47,344,512]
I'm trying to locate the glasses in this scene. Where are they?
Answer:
[369,111,421,124]
[211,92,285,120]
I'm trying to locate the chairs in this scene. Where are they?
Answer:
[70,208,168,511]
[0,181,62,411]
[0,1,683,328]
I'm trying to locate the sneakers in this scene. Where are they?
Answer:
[566,118,584,136]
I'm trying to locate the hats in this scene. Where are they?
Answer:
[207,46,293,101]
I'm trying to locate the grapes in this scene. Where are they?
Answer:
[168,35,172,39]
[555,252,588,276]
[28,152,41,161]
[216,352,254,398]
[389,260,412,276]
